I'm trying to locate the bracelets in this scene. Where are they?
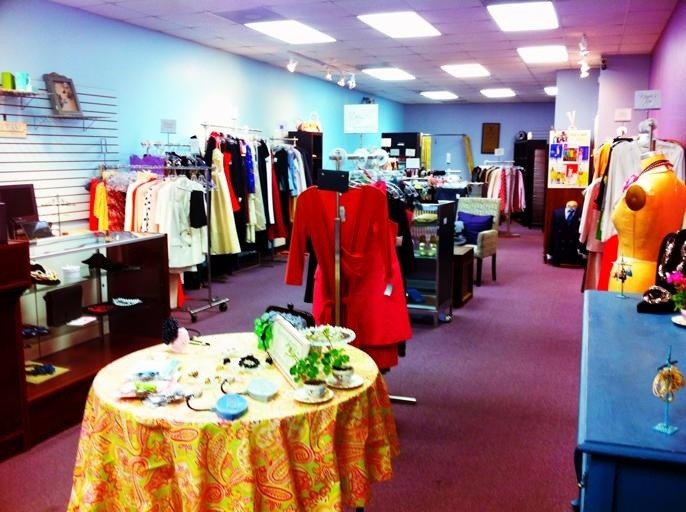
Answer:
[642,286,672,304]
[239,356,258,368]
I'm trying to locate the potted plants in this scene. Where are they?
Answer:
[320,328,354,385]
[285,345,327,399]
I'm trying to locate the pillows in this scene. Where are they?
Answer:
[459,212,494,245]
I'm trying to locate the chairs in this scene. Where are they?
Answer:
[454,197,501,287]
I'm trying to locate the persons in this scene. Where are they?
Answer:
[548,200,584,263]
[607,151,686,295]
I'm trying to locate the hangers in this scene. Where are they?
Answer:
[100,163,221,192]
[482,159,516,171]
[332,148,429,203]
[636,118,658,150]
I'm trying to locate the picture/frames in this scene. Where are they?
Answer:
[482,123,500,154]
[42,72,83,116]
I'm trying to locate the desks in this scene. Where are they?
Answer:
[432,180,470,204]
[576,288,686,512]
[64,332,402,512]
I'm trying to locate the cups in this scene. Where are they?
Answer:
[331,366,354,384]
[304,380,326,398]
[1,71,31,91]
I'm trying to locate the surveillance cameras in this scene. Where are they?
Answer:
[601,55,608,70]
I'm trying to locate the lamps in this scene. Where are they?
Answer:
[446,153,452,171]
[287,51,357,90]
[634,89,661,117]
[615,108,632,141]
[576,32,590,78]
[344,104,378,156]
[381,138,392,152]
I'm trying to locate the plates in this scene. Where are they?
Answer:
[326,375,364,390]
[293,388,334,404]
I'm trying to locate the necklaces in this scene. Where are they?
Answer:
[624,159,671,187]
[657,237,686,283]
[30,267,57,285]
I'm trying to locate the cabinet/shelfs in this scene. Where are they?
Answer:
[382,133,432,171]
[288,130,323,188]
[388,199,457,327]
[0,229,170,463]
[514,140,549,230]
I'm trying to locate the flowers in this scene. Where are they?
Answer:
[666,270,686,315]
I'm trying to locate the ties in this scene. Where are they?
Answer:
[567,209,575,225]
